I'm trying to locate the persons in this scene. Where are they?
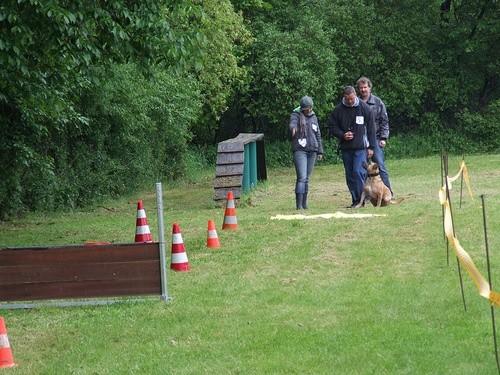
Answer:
[289,97,322,208]
[328,86,374,208]
[354,77,394,197]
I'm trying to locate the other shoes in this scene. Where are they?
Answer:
[345,203,364,210]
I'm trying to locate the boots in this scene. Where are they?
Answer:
[302,192,308,209]
[296,193,303,209]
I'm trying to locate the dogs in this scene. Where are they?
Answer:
[355,161,404,207]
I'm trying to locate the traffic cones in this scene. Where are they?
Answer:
[221,191,239,230]
[0,316,19,369]
[170,223,192,272]
[134,200,154,243]
[206,219,221,249]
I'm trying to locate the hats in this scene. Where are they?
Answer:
[300,95,313,110]
